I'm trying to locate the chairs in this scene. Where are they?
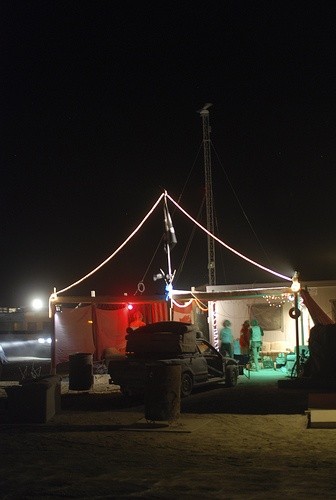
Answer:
[262,355,274,367]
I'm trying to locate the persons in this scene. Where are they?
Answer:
[240,320,251,371]
[219,320,234,358]
[130,311,146,331]
[249,319,264,372]
[124,327,134,354]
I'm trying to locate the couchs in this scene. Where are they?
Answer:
[259,342,294,361]
[287,346,309,371]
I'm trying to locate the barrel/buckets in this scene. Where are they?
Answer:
[68,352,93,390]
[145,364,181,422]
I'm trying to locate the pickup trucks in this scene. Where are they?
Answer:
[106,320,241,398]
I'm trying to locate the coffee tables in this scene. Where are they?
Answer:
[275,357,286,365]
